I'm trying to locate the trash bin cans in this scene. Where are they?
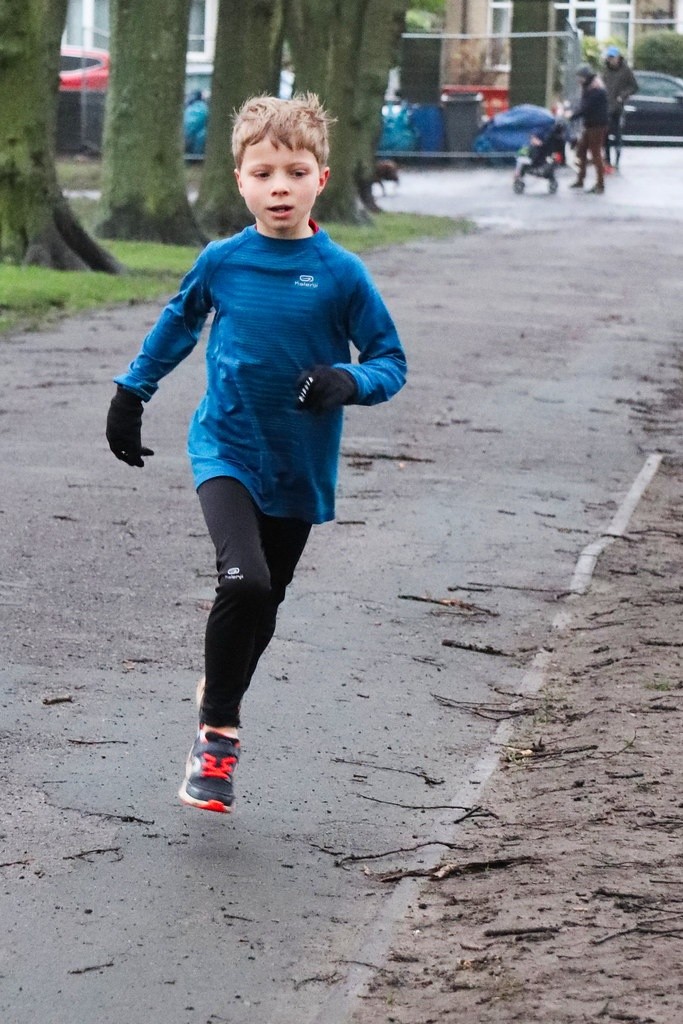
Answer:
[440,91,485,167]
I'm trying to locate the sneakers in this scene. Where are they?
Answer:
[181,728,242,814]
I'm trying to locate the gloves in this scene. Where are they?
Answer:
[105,385,155,467]
[295,366,356,416]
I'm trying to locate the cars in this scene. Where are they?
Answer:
[617,70,683,139]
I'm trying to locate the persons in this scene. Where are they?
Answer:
[105,92,414,811]
[515,131,549,185]
[598,48,643,175]
[562,65,611,195]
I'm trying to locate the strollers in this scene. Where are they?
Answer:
[512,118,575,197]
